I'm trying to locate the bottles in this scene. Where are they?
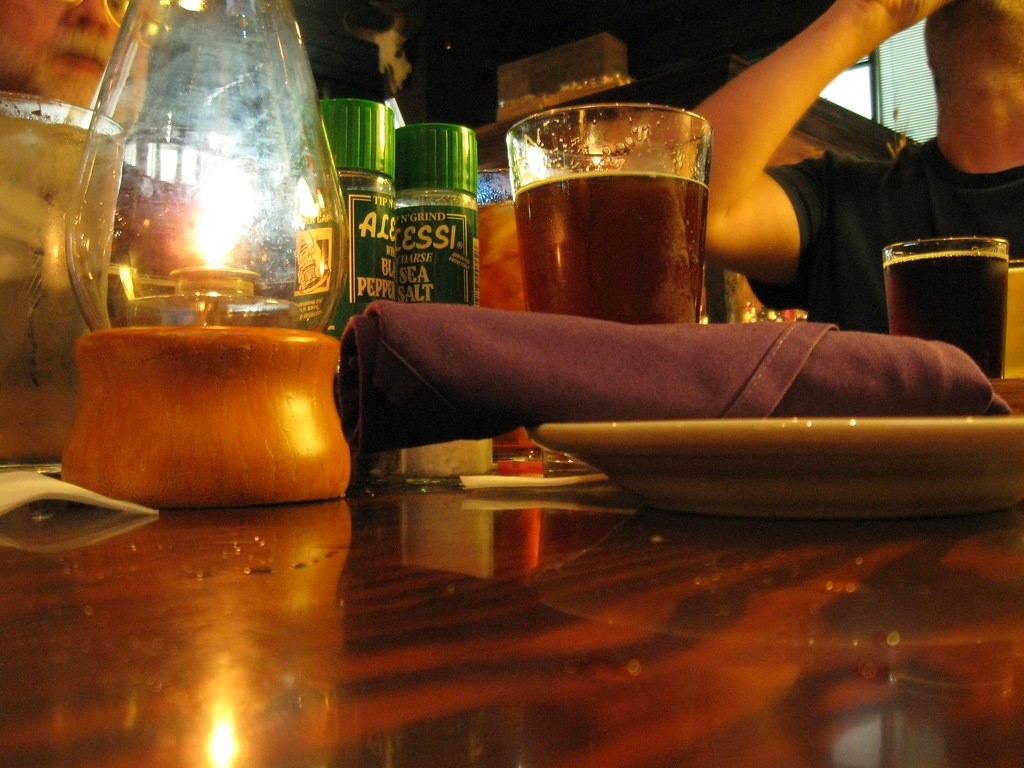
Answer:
[397,122,494,486]
[297,99,396,492]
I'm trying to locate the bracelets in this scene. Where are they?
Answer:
[25,287,44,389]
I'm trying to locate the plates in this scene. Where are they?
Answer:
[538,416,1024,521]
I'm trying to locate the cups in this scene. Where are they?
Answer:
[476,169,546,461]
[505,103,715,324]
[1002,259,1024,380]
[0,92,126,473]
[883,237,1011,380]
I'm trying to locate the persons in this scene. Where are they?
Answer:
[0,0,258,464]
[681,0,1024,337]
